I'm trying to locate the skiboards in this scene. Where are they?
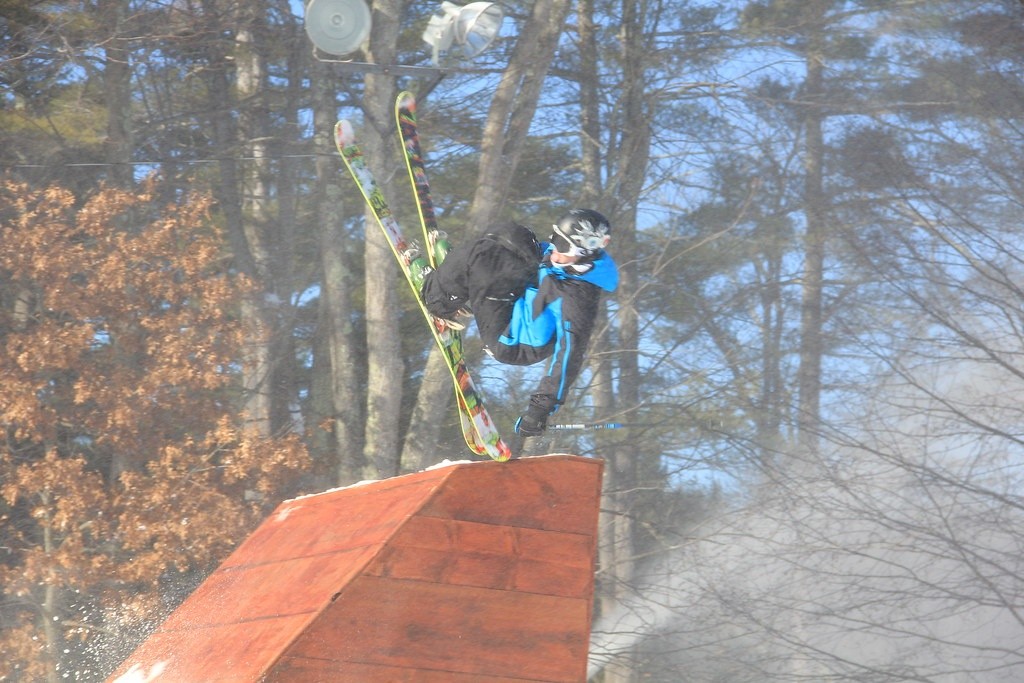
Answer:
[330,87,514,464]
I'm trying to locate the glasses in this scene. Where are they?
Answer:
[551,232,571,253]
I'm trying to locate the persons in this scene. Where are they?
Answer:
[402,208,611,437]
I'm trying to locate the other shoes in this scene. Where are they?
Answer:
[411,258,434,294]
[435,240,453,269]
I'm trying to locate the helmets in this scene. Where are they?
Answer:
[550,209,613,274]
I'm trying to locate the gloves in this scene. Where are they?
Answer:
[519,405,550,437]
